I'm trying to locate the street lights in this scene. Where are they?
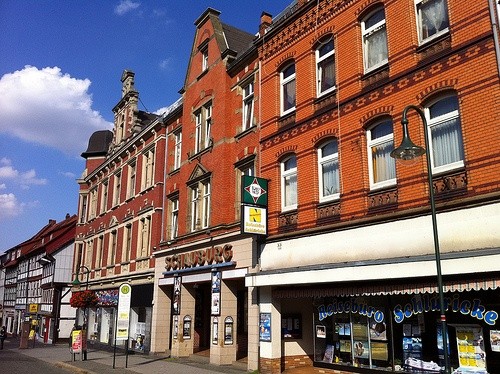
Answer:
[390,104,451,372]
[73,266,88,361]
